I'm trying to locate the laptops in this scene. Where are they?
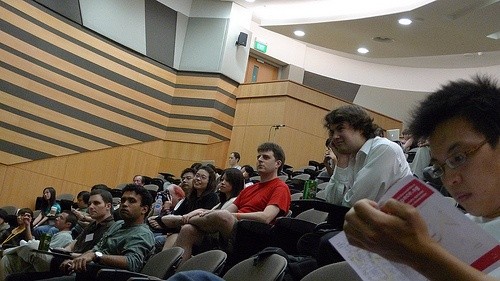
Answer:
[386,129,400,141]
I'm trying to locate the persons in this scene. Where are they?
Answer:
[317,75,500,281]
[0,142,291,281]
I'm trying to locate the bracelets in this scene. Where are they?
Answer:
[81,216,86,221]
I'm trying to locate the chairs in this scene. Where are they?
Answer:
[0,159,363,281]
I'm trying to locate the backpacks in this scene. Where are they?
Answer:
[258,246,319,281]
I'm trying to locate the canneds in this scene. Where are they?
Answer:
[38,233,52,252]
[303,179,317,199]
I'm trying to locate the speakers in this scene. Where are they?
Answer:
[237,32,248,46]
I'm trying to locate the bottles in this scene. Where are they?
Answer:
[153,196,162,216]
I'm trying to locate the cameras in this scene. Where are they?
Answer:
[161,189,170,203]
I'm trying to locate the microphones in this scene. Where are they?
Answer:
[272,125,284,127]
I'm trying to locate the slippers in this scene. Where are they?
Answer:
[146,215,180,233]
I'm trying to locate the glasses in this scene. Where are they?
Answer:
[195,174,209,182]
[427,137,489,179]
[180,176,194,182]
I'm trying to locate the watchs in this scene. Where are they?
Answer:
[95,251,103,263]
